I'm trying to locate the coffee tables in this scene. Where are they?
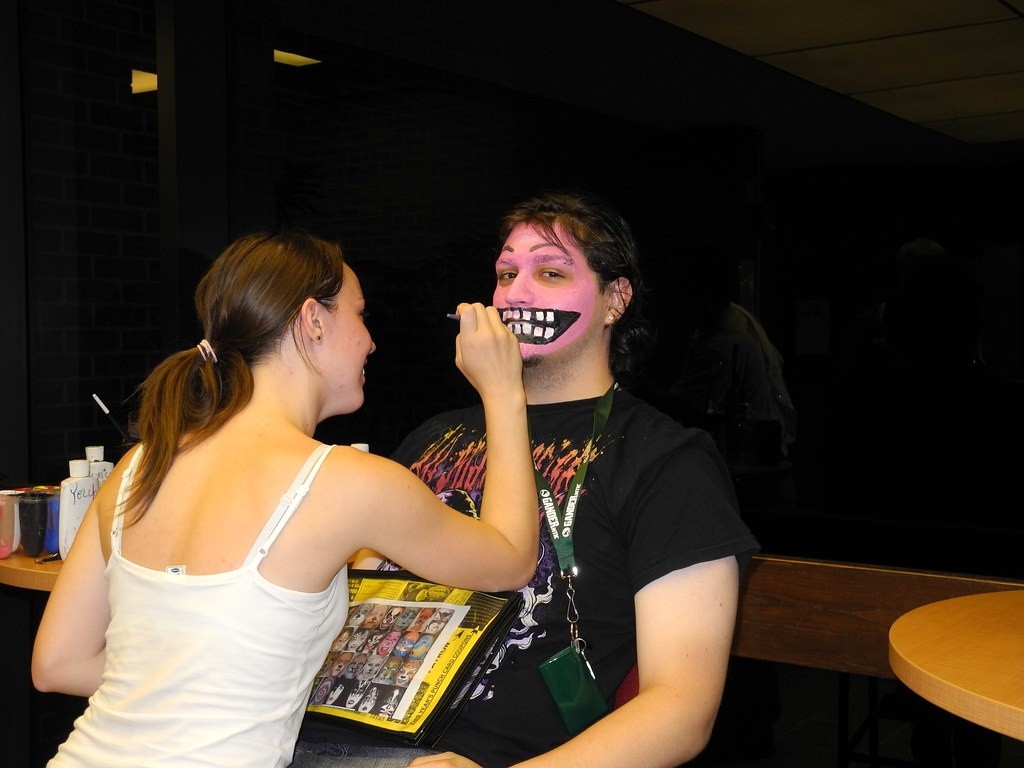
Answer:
[0,543,69,591]
[886,589,1024,741]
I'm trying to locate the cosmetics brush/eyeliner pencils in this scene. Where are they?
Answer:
[446,311,462,323]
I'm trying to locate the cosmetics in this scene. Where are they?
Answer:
[0,443,113,561]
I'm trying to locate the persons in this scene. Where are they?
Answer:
[28,233,543,768]
[374,190,755,768]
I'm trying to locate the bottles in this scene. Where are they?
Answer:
[84,445,115,485]
[58,460,98,562]
